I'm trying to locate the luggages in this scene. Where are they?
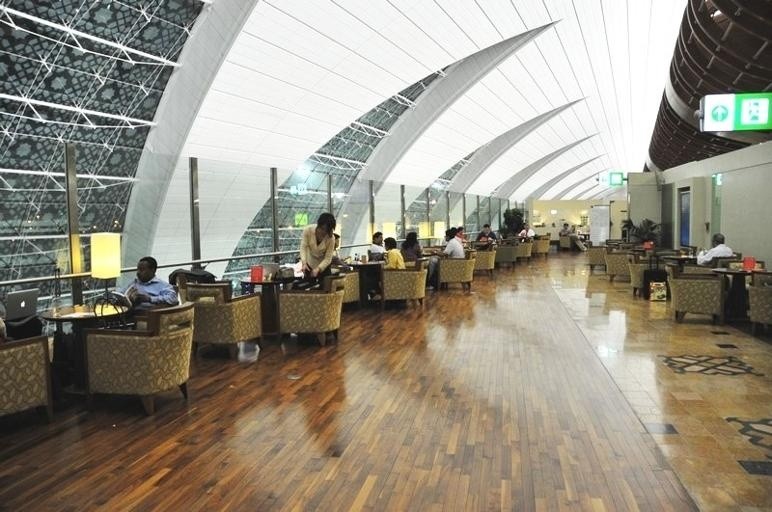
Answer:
[643,254,667,300]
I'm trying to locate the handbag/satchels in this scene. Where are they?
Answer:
[649,281,667,301]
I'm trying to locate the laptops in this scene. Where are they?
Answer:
[0,288,39,322]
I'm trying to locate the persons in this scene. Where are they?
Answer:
[331,234,347,267]
[427,224,495,280]
[373,237,405,300]
[518,223,535,242]
[400,232,423,262]
[559,224,571,237]
[112,256,178,312]
[299,212,335,281]
[370,232,388,258]
[697,234,733,266]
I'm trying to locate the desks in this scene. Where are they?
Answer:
[241,273,297,336]
[39,302,128,394]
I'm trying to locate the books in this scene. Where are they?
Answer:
[111,284,139,307]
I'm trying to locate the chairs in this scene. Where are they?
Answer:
[84,319,193,415]
[191,291,263,352]
[149,302,198,330]
[275,281,346,351]
[332,230,550,309]
[0,330,54,429]
[176,270,232,304]
[560,229,771,344]
